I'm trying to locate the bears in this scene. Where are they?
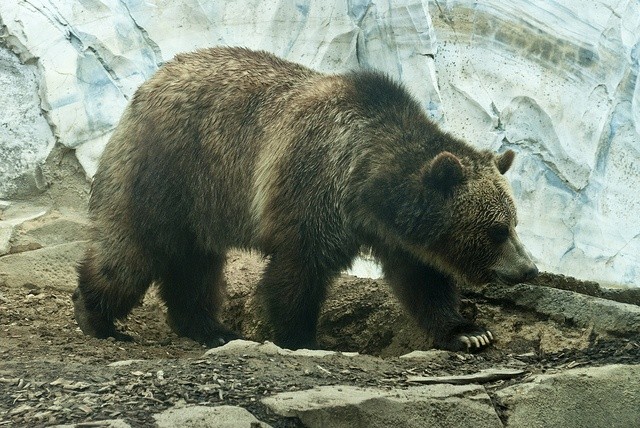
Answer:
[72,46,537,354]
